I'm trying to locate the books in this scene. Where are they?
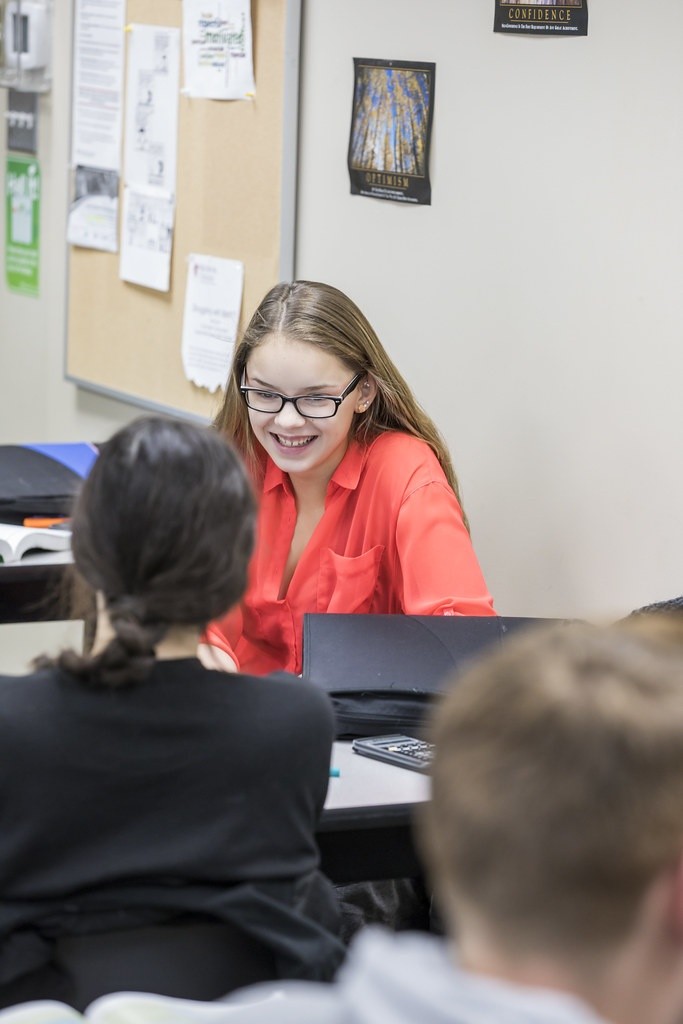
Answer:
[0,523,75,563]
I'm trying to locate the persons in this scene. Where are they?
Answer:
[0,416,361,1012]
[192,281,496,887]
[233,614,683,1024]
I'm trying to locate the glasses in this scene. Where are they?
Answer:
[240,365,366,420]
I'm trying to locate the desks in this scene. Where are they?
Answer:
[0,615,436,831]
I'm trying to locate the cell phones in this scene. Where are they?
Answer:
[352,734,438,774]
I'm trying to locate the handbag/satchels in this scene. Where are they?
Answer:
[303,612,569,743]
